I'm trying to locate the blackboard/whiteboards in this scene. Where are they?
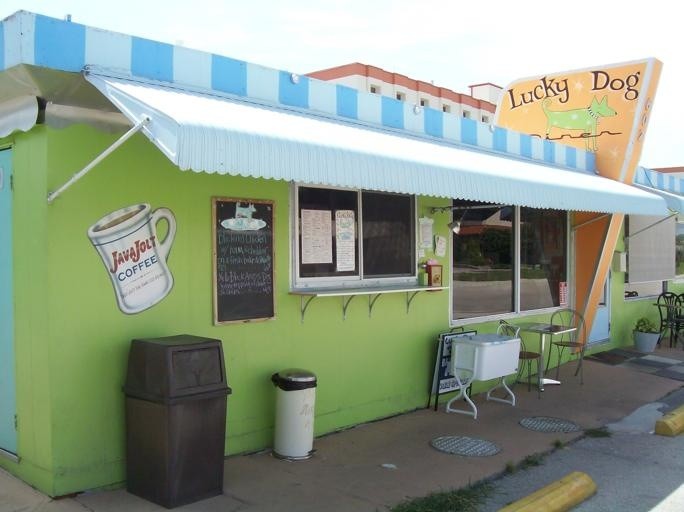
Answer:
[210,195,277,326]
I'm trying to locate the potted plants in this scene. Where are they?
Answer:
[629,317,662,353]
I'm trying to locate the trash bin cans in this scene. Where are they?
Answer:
[273,368,318,459]
[120,333,233,509]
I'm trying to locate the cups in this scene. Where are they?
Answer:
[87,203,177,317]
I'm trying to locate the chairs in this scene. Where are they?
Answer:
[652,291,683,349]
[498,308,588,402]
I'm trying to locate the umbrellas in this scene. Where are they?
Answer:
[480,207,526,227]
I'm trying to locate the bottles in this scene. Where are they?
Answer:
[418,264,429,287]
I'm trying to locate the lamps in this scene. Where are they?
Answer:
[429,205,471,236]
